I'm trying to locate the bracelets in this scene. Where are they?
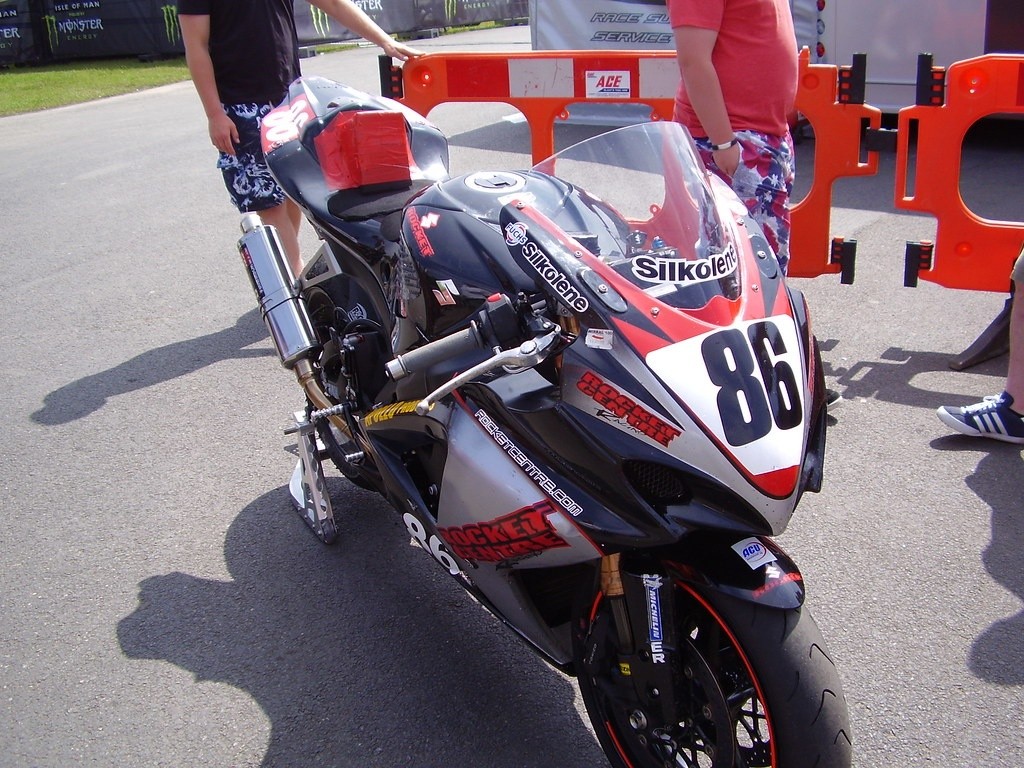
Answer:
[711,137,737,150]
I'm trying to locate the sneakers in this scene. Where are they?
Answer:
[936,390,1023,443]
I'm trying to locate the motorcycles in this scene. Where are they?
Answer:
[235,74,854,768]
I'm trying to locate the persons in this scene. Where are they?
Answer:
[174,0,427,281]
[665,0,845,413]
[935,248,1024,444]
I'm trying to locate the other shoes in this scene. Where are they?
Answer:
[827,389,843,411]
